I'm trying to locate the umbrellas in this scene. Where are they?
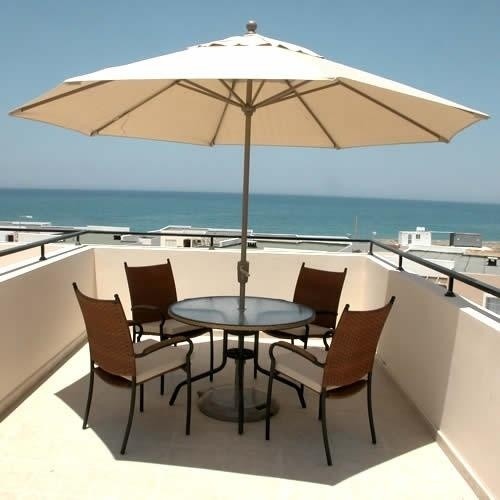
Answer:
[7,20,490,388]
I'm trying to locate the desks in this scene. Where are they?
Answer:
[168,294,315,434]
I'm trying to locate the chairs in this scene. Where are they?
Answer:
[253,262,347,392]
[72,282,194,455]
[265,295,396,466]
[123,257,213,395]
